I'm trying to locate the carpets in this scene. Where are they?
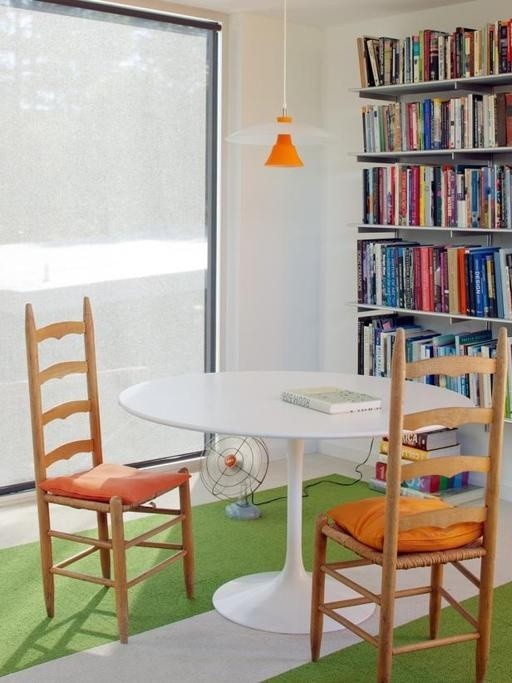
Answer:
[2,475,401,676]
[266,585,508,682]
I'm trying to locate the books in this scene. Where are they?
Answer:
[368,428,484,507]
[362,162,511,229]
[357,316,498,408]
[363,93,512,152]
[281,386,382,415]
[356,18,512,88]
[355,238,511,320]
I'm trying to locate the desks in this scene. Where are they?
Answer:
[118,370,473,636]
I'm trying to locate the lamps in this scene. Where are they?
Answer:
[226,1,332,170]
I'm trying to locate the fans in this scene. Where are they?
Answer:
[199,434,269,520]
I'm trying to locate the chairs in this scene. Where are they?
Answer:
[25,296,194,645]
[309,325,508,683]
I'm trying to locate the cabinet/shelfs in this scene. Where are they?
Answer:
[349,70,512,422]
